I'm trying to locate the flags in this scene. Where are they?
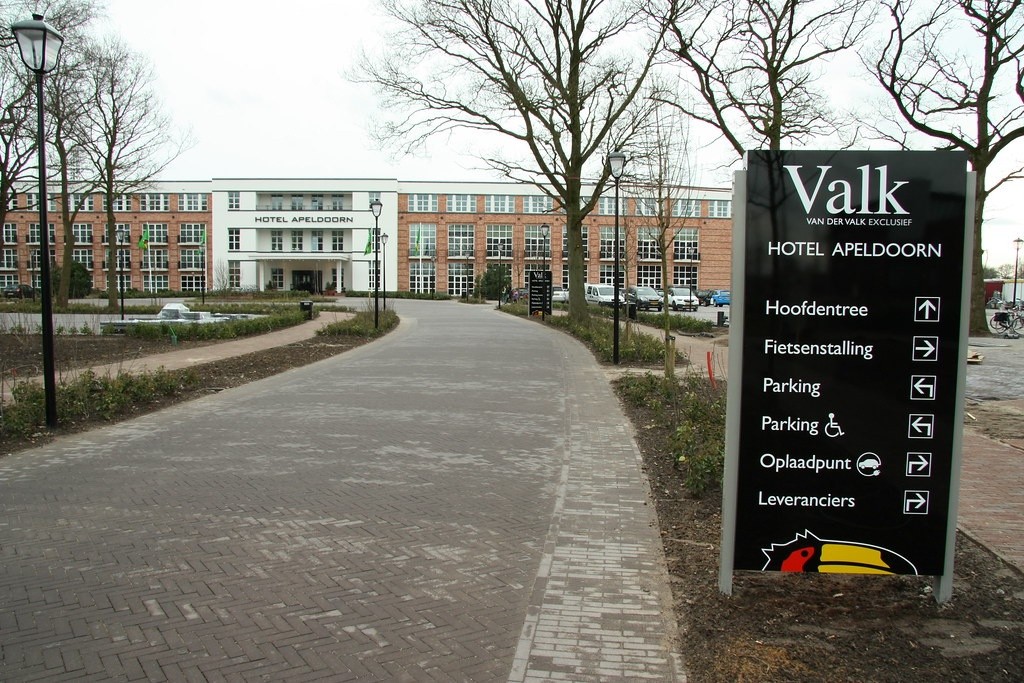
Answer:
[363,231,371,255]
[137,226,148,249]
[200,230,205,246]
[415,228,420,256]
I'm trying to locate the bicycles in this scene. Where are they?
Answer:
[990,306,1024,335]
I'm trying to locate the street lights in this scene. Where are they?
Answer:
[431,257,435,299]
[608,148,626,365]
[465,252,470,301]
[117,229,126,321]
[381,233,388,310]
[540,222,549,321]
[199,244,205,304]
[689,248,694,312]
[1013,238,1024,307]
[498,243,503,309]
[10,14,64,426]
[29,247,36,302]
[371,199,383,328]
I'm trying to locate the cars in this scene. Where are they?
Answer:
[667,288,699,312]
[621,287,662,312]
[655,290,664,304]
[565,283,587,304]
[3,284,34,299]
[695,290,708,305]
[987,297,1003,309]
[505,288,528,303]
[586,285,625,308]
[1015,300,1024,311]
[547,286,566,303]
[703,290,730,307]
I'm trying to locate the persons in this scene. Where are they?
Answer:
[504,285,519,304]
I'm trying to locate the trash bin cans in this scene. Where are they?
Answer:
[300,300,313,321]
[622,302,636,320]
[462,290,466,297]
